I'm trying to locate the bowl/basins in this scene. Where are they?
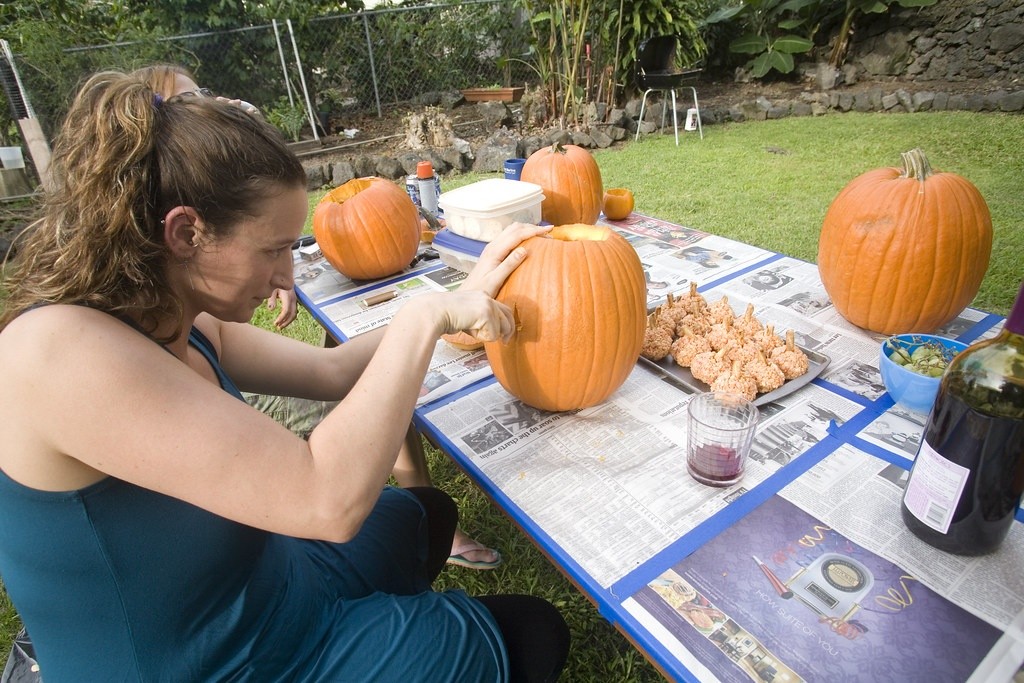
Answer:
[879,333,973,414]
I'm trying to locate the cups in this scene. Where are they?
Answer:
[686,393,760,487]
[504,158,526,181]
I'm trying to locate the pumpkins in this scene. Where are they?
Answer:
[818,148,993,336]
[519,141,603,226]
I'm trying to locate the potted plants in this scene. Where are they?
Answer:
[463,83,525,103]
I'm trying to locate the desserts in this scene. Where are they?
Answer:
[639,281,810,406]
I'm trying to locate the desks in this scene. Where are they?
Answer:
[291,201,1023,683]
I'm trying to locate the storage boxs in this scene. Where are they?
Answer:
[431,221,551,274]
[438,178,546,244]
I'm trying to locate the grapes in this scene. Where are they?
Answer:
[884,336,953,378]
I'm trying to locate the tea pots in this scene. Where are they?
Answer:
[406,168,441,208]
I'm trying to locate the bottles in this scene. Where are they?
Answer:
[417,161,439,219]
[900,278,1024,556]
[685,104,698,131]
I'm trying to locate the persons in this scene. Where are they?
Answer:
[0,64,571,683]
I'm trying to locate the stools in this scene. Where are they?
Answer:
[633,86,703,146]
